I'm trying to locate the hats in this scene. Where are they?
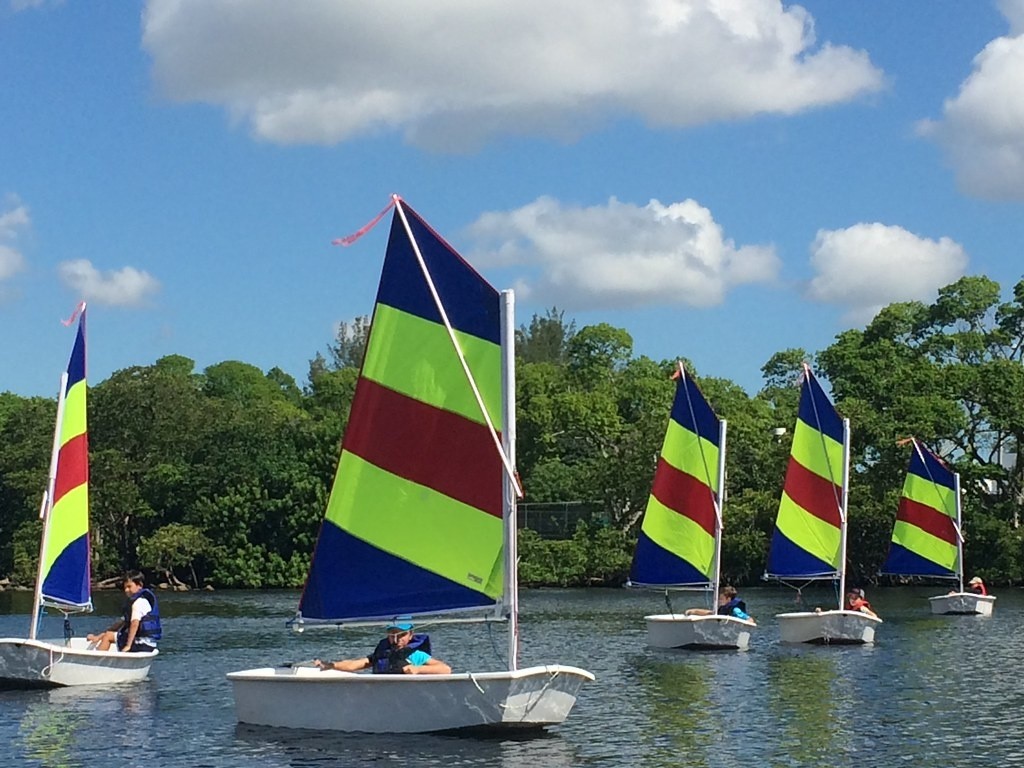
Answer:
[969,577,982,584]
[386,615,413,630]
[847,588,864,599]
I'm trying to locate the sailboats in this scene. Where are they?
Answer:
[623,359,774,654]
[218,191,616,731]
[1,301,163,683]
[889,432,1006,616]
[747,361,896,654]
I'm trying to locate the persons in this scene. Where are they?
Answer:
[816,588,883,619]
[947,576,987,598]
[685,586,755,625]
[87,569,162,654]
[312,615,451,675]
